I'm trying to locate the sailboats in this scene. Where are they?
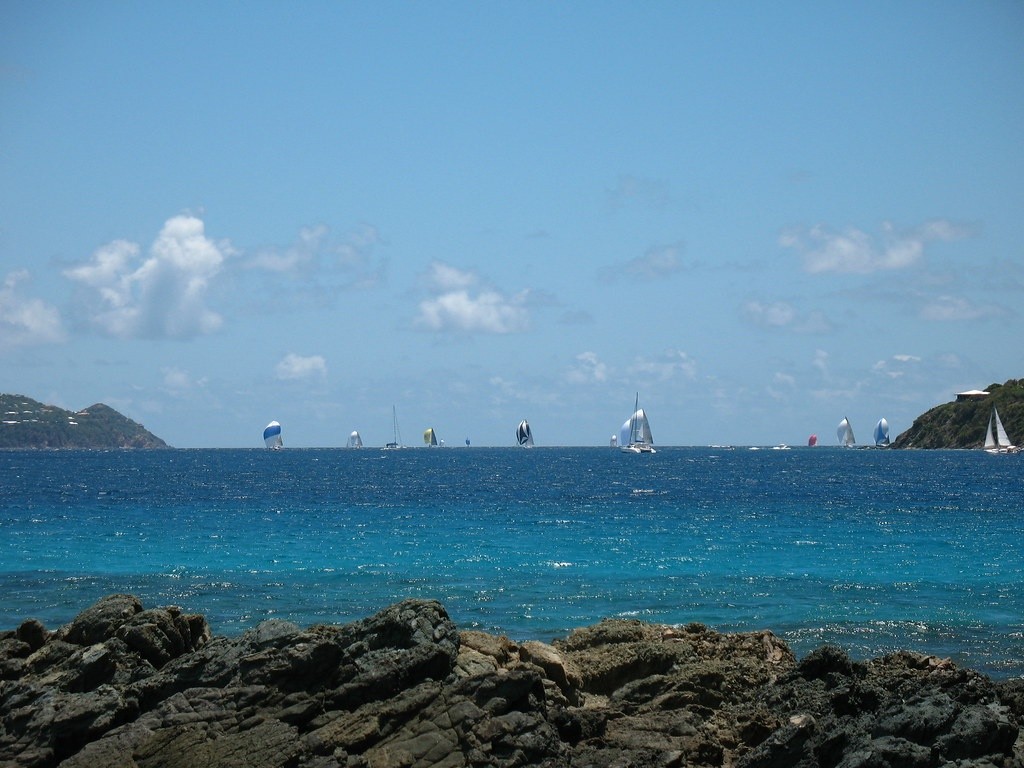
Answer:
[380,405,407,450]
[424,428,445,448]
[837,416,856,447]
[809,433,818,447]
[516,418,535,449]
[873,417,891,450]
[465,438,470,448]
[982,402,1021,454]
[620,392,657,454]
[263,420,284,451]
[346,430,363,451]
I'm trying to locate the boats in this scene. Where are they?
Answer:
[773,445,792,450]
[749,446,760,450]
